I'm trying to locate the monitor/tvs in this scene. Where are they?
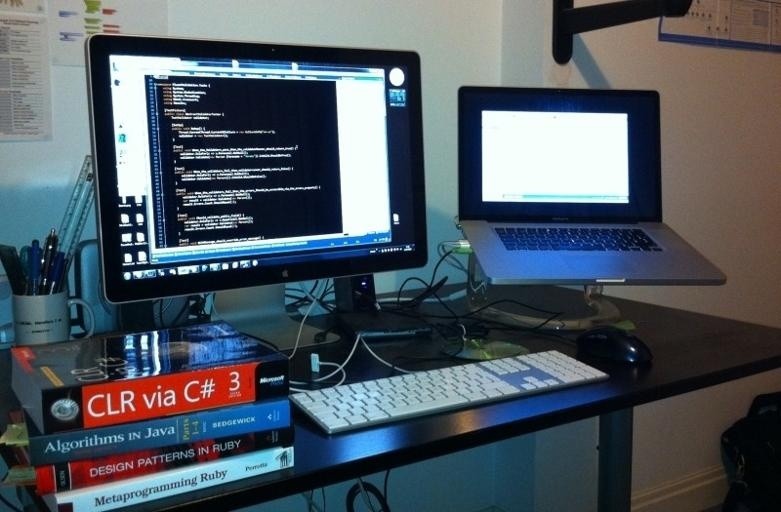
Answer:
[85,35,430,354]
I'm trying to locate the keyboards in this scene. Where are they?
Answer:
[289,348,609,438]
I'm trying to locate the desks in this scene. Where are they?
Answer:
[1,281,780,512]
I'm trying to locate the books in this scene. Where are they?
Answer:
[42,445,295,512]
[12,425,297,496]
[12,397,291,468]
[10,321,291,436]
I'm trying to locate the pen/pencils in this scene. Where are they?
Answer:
[2,227,65,295]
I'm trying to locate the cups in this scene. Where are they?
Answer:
[12,291,96,346]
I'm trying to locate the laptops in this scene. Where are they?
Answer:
[455,84,729,285]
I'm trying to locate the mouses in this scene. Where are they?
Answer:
[577,325,655,363]
